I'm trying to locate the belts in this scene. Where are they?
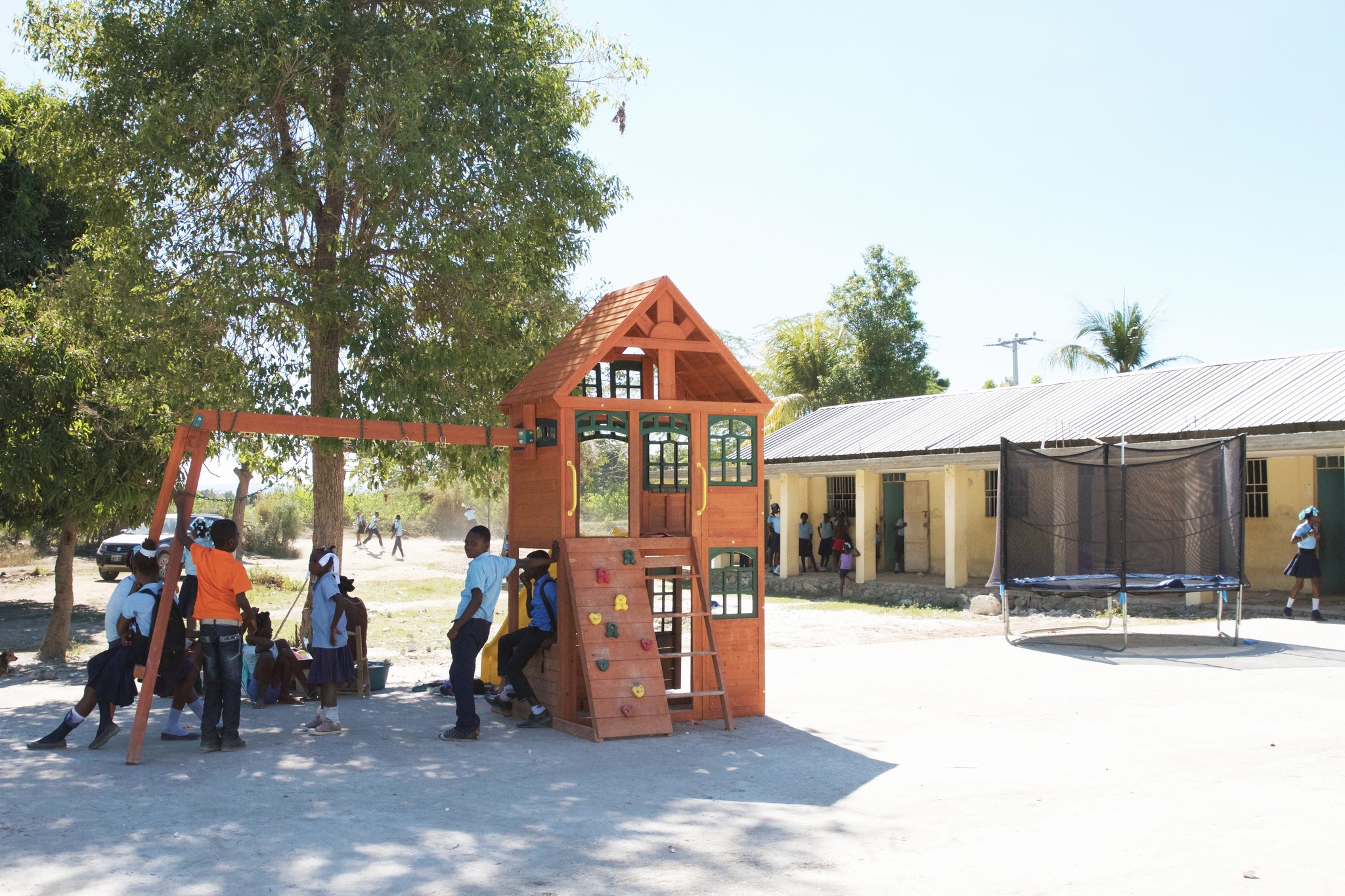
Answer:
[202,619,241,627]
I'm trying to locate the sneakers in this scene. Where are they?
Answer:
[308,717,342,736]
[1283,607,1294,618]
[440,726,478,741]
[300,715,325,731]
[1311,609,1327,621]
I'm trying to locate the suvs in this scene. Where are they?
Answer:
[95,514,226,584]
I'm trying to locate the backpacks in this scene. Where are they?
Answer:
[392,521,400,534]
[135,588,186,661]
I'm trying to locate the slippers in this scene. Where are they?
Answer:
[300,694,321,701]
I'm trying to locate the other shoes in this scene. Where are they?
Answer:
[769,570,773,573]
[26,737,67,750]
[516,710,551,728]
[355,543,361,547]
[201,738,222,752]
[773,571,779,576]
[88,723,120,750]
[815,569,822,572]
[222,738,246,751]
[484,694,513,711]
[162,731,200,740]
[895,570,902,573]
[800,570,807,573]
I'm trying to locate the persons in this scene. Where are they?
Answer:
[439,525,561,742]
[333,574,368,691]
[836,542,861,601]
[461,507,477,542]
[299,545,355,736]
[1282,505,1328,623]
[352,511,365,547]
[27,517,321,752]
[362,510,386,549]
[390,515,407,558]
[766,503,907,577]
[504,534,509,582]
[484,549,558,728]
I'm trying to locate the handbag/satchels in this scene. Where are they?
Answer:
[365,523,371,534]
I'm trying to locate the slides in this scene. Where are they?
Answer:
[480,527,630,685]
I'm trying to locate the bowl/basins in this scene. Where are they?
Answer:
[350,661,394,691]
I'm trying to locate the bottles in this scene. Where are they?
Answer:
[1243,639,1258,645]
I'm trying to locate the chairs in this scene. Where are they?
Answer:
[335,626,371,698]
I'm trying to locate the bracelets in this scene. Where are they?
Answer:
[1300,536,1303,541]
[1306,534,1309,537]
